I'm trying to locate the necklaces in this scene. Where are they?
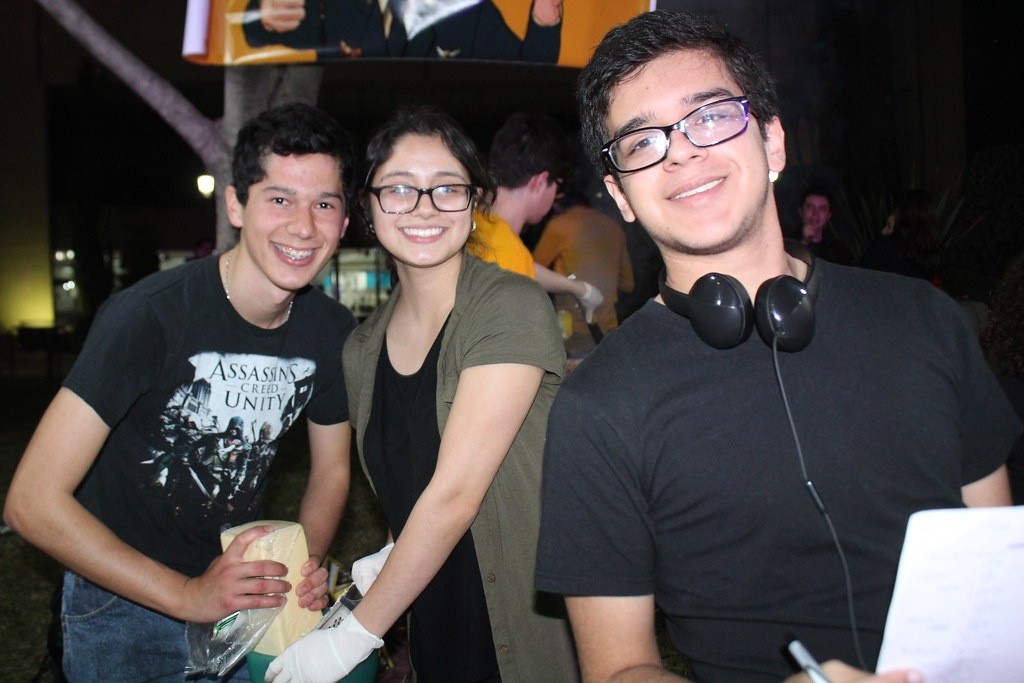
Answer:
[225,250,293,321]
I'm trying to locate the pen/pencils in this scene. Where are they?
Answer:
[786,638,834,683]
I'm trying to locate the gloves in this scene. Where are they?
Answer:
[351,543,395,596]
[264,611,384,683]
[576,281,604,324]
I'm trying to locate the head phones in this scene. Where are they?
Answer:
[658,237,820,353]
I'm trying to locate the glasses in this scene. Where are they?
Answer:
[368,184,478,214]
[601,95,769,174]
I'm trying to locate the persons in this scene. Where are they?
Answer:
[532,175,635,377]
[3,102,358,683]
[342,109,582,683]
[533,7,1024,683]
[879,197,942,291]
[793,186,852,267]
[241,0,563,65]
[463,114,603,323]
